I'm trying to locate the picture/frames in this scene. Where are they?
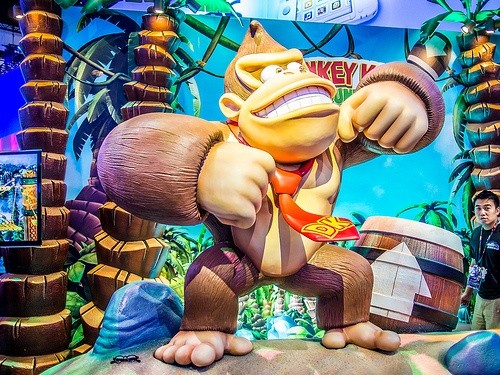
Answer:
[0,149,42,249]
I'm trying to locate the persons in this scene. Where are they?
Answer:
[460,189,500,331]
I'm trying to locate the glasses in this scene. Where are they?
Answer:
[110,355,141,364]
